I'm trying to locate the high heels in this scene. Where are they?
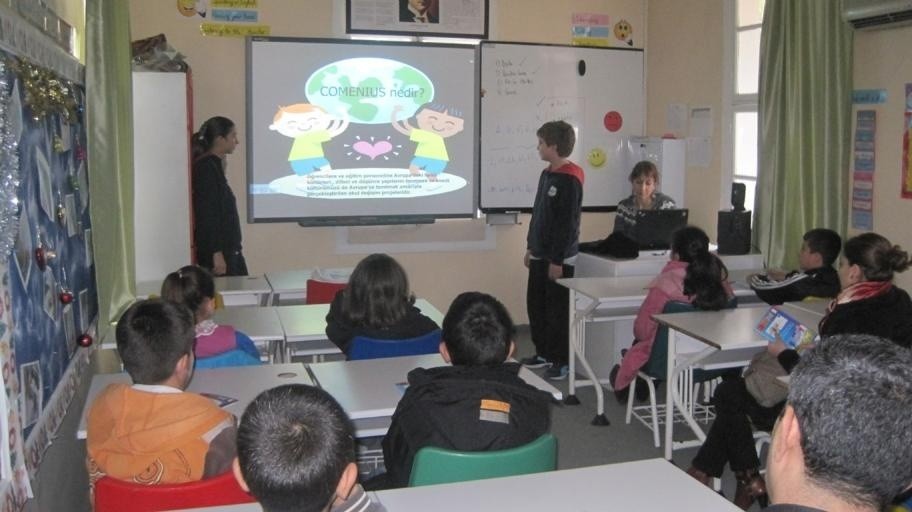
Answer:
[734,466,767,510]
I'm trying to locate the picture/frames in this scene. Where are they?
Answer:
[345,0,490,40]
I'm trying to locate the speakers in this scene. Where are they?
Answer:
[731,183,746,212]
[718,211,751,255]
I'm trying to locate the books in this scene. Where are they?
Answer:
[753,306,811,350]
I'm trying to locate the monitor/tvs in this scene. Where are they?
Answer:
[636,209,688,250]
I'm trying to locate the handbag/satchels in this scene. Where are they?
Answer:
[744,352,789,408]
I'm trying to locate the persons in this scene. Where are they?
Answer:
[516,120,585,381]
[753,331,912,512]
[160,263,262,367]
[233,383,389,512]
[402,1,441,24]
[608,225,738,407]
[362,290,555,494]
[325,251,440,362]
[687,231,912,512]
[612,160,677,238]
[746,227,843,307]
[191,116,249,277]
[86,292,240,509]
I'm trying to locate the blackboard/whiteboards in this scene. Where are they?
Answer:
[480,42,648,212]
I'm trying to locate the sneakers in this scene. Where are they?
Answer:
[520,356,569,380]
